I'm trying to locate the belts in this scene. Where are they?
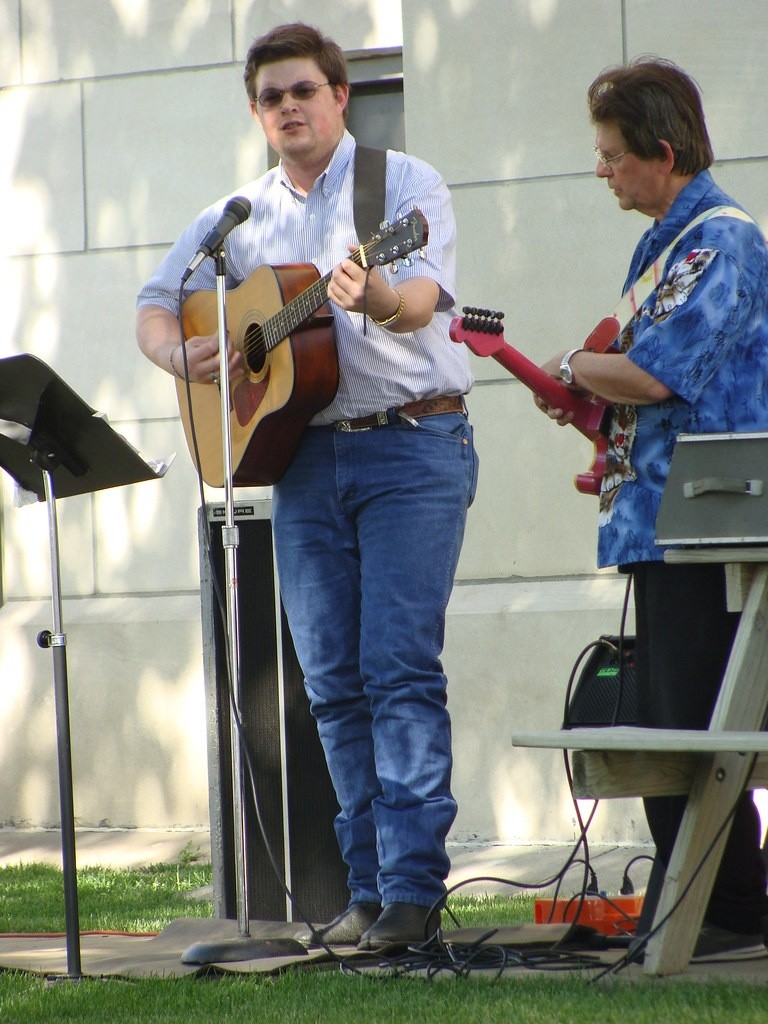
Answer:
[305,393,464,433]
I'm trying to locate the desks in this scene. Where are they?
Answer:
[642,545,768,979]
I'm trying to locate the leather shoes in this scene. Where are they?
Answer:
[357,901,441,950]
[294,901,383,948]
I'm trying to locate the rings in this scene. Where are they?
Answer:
[211,370,218,383]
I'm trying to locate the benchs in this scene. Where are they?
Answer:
[513,725,768,753]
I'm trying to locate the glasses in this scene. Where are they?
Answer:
[254,81,332,107]
[594,150,631,170]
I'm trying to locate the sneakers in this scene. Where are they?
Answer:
[628,923,767,962]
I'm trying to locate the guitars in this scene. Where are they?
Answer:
[447,306,624,494]
[172,205,428,491]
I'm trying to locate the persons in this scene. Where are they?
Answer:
[136,23,479,951]
[534,53,767,965]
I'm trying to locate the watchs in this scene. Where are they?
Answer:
[559,349,585,384]
[369,288,405,327]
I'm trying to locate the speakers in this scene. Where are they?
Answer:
[197,497,351,923]
[564,635,639,725]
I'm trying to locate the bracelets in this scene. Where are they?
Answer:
[169,347,196,383]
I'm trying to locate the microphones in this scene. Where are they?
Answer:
[181,197,252,282]
